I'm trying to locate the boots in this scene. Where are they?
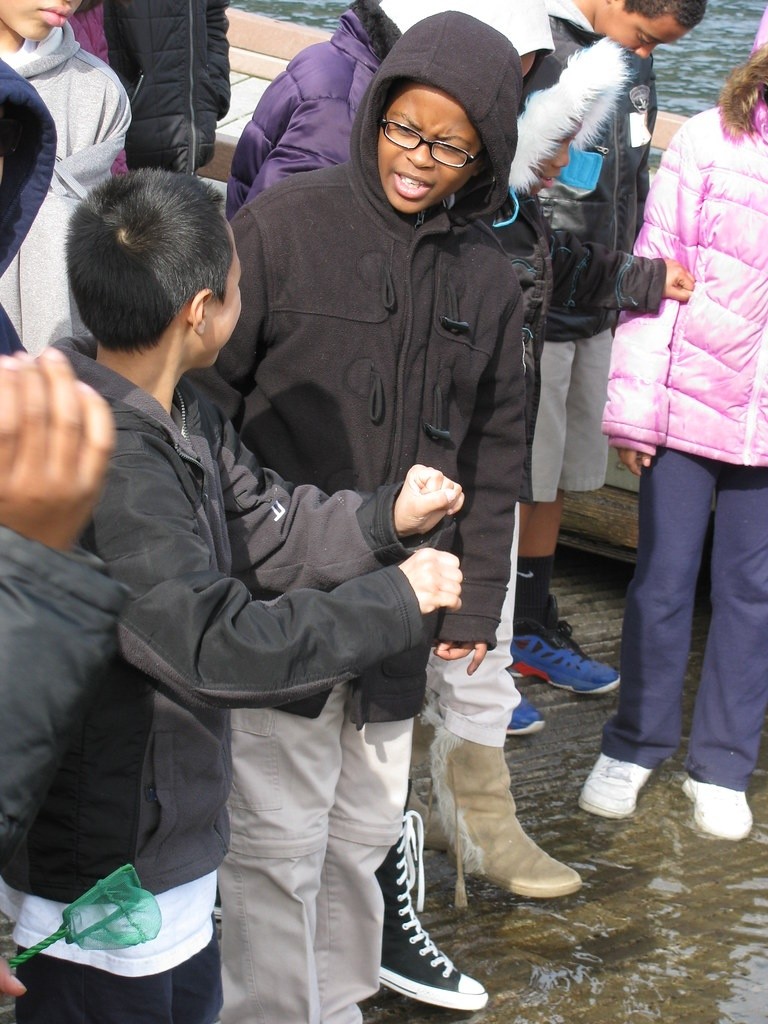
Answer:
[406,686,453,852]
[374,779,488,1011]
[429,725,583,898]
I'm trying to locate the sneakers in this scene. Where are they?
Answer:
[506,594,621,695]
[681,777,754,841]
[506,695,546,736]
[578,752,653,819]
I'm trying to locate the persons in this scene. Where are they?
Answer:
[0,0,704,1023]
[577,0,767,839]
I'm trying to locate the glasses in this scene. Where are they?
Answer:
[382,115,484,169]
